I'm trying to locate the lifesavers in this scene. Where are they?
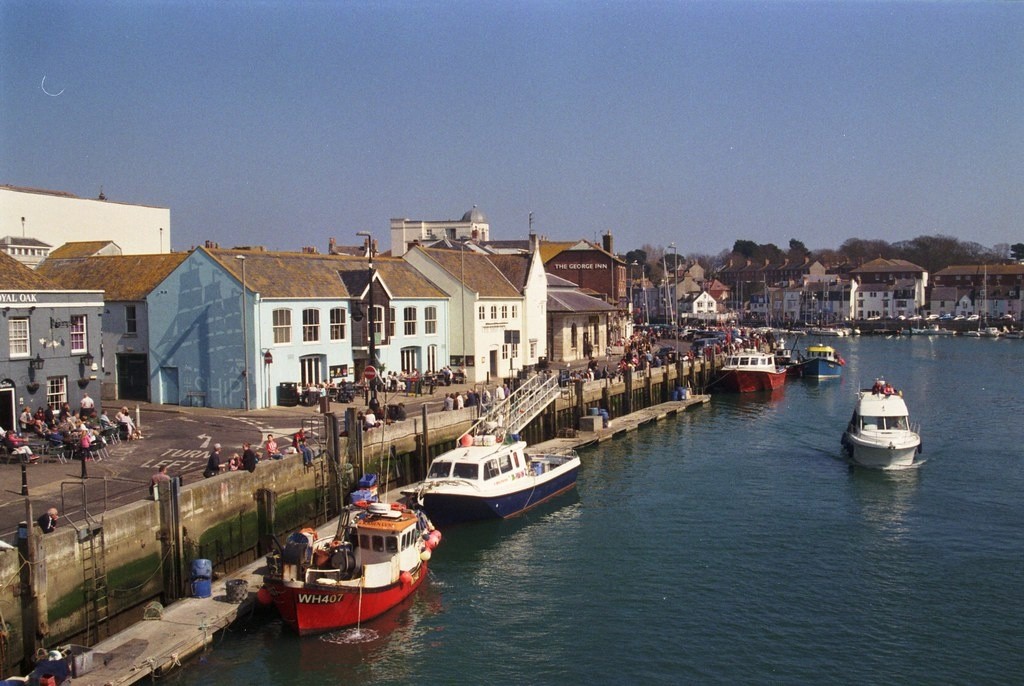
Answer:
[389,502,406,511]
[352,500,376,508]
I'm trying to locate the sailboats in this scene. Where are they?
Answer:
[872,263,1024,340]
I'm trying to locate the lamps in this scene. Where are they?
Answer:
[80,351,94,366]
[30,353,44,370]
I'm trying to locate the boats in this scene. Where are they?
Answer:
[785,309,846,381]
[251,472,442,640]
[413,417,582,530]
[769,322,862,379]
[717,343,789,395]
[840,379,923,469]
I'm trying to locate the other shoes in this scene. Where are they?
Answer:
[84,457,91,462]
[30,455,39,460]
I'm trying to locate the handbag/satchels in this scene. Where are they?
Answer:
[203,468,217,479]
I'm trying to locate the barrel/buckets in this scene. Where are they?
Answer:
[671,386,692,400]
[0,652,67,686]
[190,559,212,597]
[588,407,609,421]
[283,532,309,564]
[530,461,543,475]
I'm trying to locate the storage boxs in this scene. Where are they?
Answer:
[59,644,93,677]
[360,473,378,501]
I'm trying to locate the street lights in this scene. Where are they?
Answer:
[235,255,250,411]
[629,261,639,324]
[667,245,679,362]
[350,231,376,400]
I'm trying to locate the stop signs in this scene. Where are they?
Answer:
[364,365,377,381]
[264,352,273,364]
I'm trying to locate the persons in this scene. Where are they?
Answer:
[48,413,106,462]
[207,433,281,476]
[670,324,775,355]
[79,392,94,417]
[101,406,144,439]
[872,382,895,395]
[0,427,39,465]
[292,429,314,467]
[357,397,407,433]
[71,409,86,428]
[88,408,101,425]
[297,364,467,404]
[441,383,510,410]
[20,402,71,439]
[149,465,170,496]
[37,508,58,533]
[571,328,739,383]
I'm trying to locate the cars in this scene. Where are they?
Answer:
[866,315,880,320]
[924,314,940,321]
[906,314,924,322]
[981,312,998,320]
[892,315,907,322]
[632,322,753,365]
[938,313,957,321]
[1000,314,1012,320]
[967,314,981,322]
[953,314,967,321]
[880,314,893,321]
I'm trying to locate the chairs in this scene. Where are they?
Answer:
[0,418,137,464]
[299,373,466,406]
